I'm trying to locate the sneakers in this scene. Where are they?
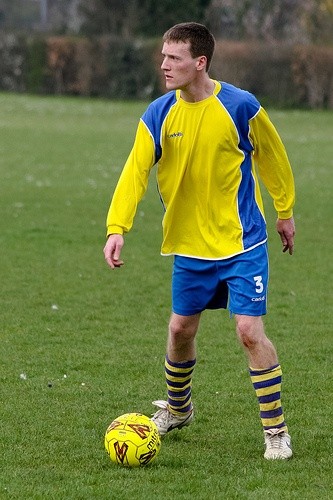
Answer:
[150,400,193,437]
[264,429,293,460]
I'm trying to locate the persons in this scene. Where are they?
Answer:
[102,22,297,461]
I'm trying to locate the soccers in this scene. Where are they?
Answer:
[104,411,162,469]
